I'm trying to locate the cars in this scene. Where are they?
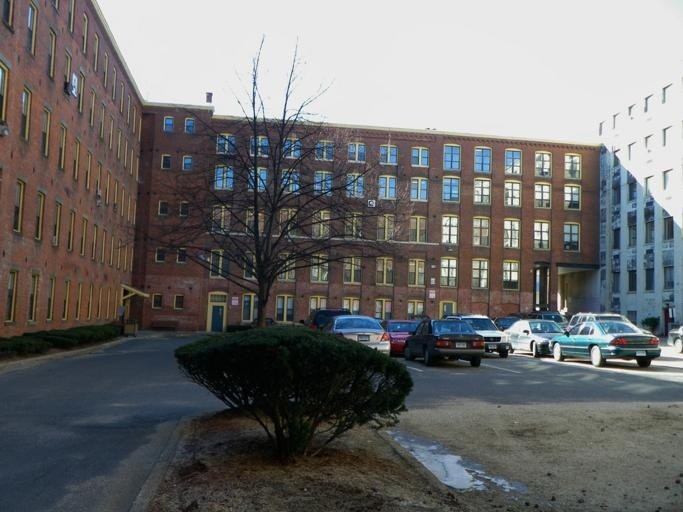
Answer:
[666,324,683,354]
